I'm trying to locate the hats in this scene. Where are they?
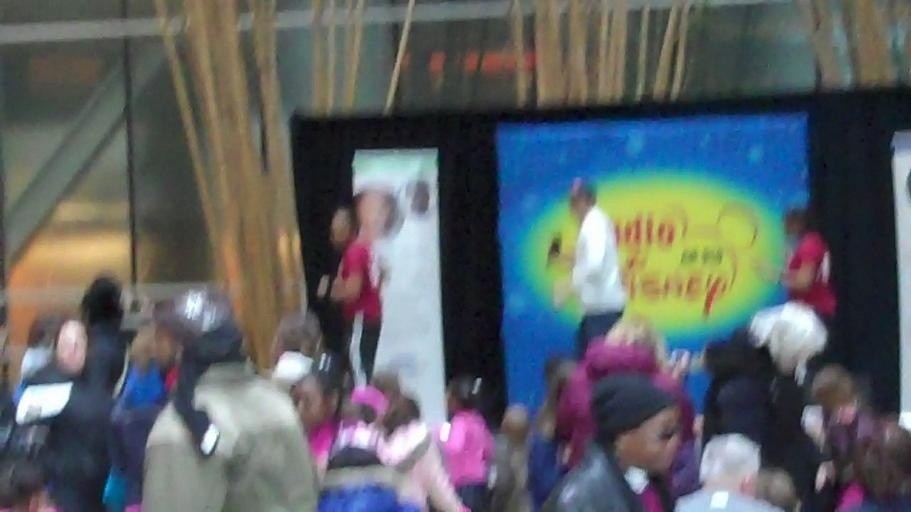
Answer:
[589,369,679,445]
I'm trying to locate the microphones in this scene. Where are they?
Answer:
[546,234,562,264]
[323,266,338,302]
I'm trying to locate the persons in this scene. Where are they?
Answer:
[1,273,534,511]
[554,179,626,360]
[528,305,910,511]
[317,207,382,394]
[779,207,837,404]
